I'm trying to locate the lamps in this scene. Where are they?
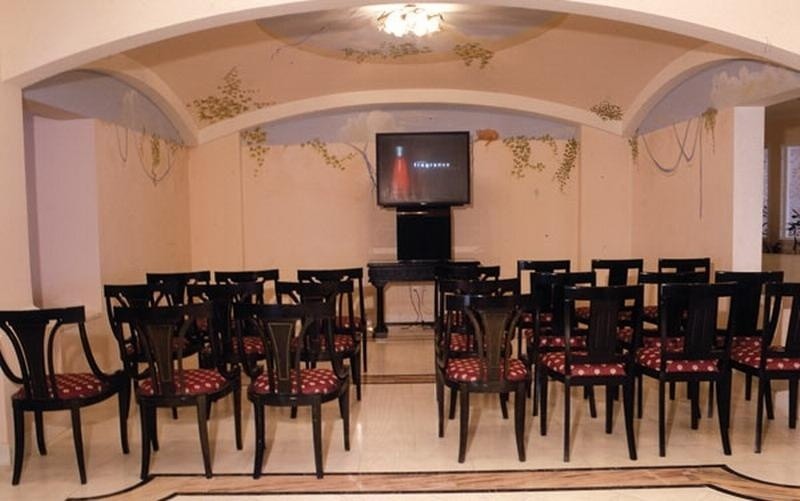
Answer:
[375,5,445,38]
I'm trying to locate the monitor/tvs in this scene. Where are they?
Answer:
[375,131,471,212]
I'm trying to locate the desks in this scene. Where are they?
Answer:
[367,261,480,340]
